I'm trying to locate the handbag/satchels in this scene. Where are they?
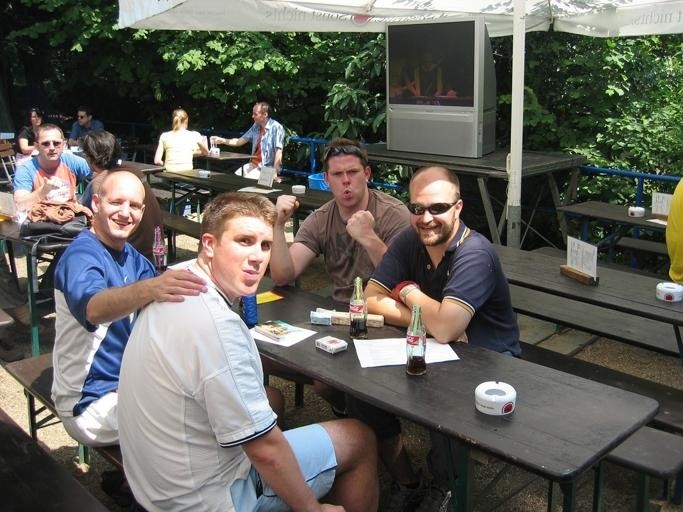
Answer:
[19,214,89,245]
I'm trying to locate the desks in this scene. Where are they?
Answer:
[360,142,586,248]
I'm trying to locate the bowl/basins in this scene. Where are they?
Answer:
[308,174,330,192]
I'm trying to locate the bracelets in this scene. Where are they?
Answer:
[388,280,421,300]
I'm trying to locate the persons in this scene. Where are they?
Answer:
[361,163,523,511]
[116,190,382,510]
[208,102,286,180]
[0,123,90,343]
[66,106,105,145]
[13,104,46,166]
[47,168,208,506]
[73,129,167,268]
[151,109,210,171]
[665,177,682,285]
[405,50,445,106]
[269,138,411,419]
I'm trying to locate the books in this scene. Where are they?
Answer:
[253,319,295,343]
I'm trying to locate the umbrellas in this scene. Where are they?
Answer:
[112,2,682,248]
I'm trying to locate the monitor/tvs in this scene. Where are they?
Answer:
[385,16,496,111]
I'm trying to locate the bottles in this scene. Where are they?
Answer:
[406,304,427,374]
[348,277,366,339]
[152,230,167,274]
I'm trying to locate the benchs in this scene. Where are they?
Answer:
[1,410,109,511]
[226,236,683,511]
[0,353,125,467]
[0,130,337,358]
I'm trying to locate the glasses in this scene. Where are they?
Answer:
[323,144,363,161]
[37,139,66,149]
[407,201,456,216]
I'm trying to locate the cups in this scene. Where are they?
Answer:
[63,138,84,154]
[208,135,220,156]
